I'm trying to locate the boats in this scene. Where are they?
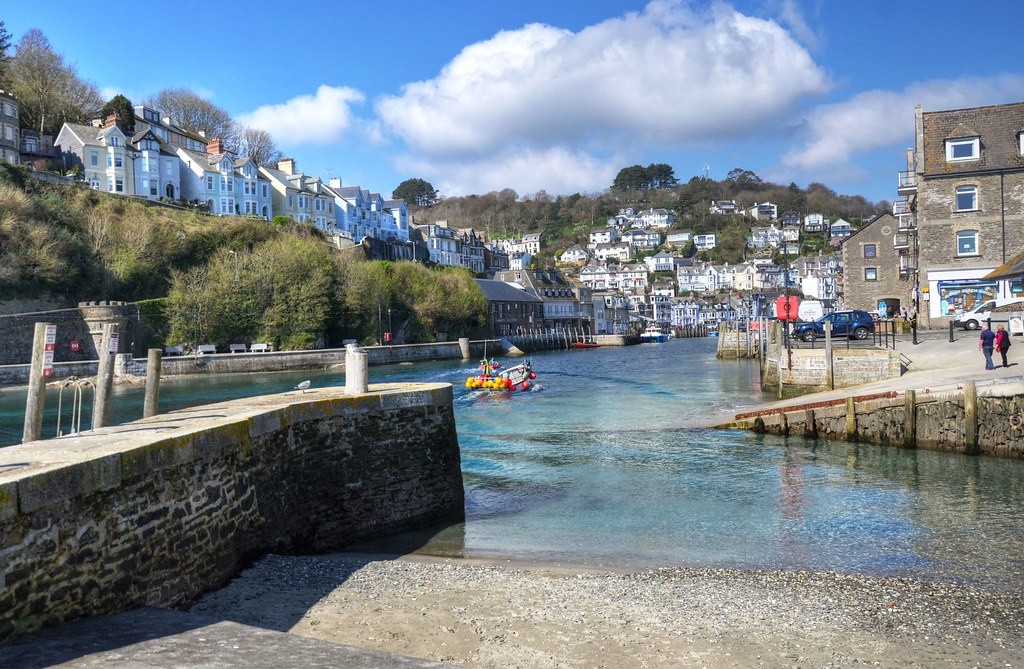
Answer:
[576,341,601,347]
[471,358,532,394]
[479,339,502,371]
[639,327,668,343]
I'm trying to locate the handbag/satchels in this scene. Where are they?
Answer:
[996,346,1001,353]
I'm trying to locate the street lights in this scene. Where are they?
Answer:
[910,269,920,344]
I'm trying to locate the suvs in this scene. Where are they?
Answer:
[791,309,876,342]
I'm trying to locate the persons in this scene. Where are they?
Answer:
[978,323,1011,370]
[901,307,907,320]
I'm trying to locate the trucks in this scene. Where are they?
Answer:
[953,296,1024,330]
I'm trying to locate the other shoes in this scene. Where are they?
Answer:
[987,368,995,371]
[1002,365,1008,368]
